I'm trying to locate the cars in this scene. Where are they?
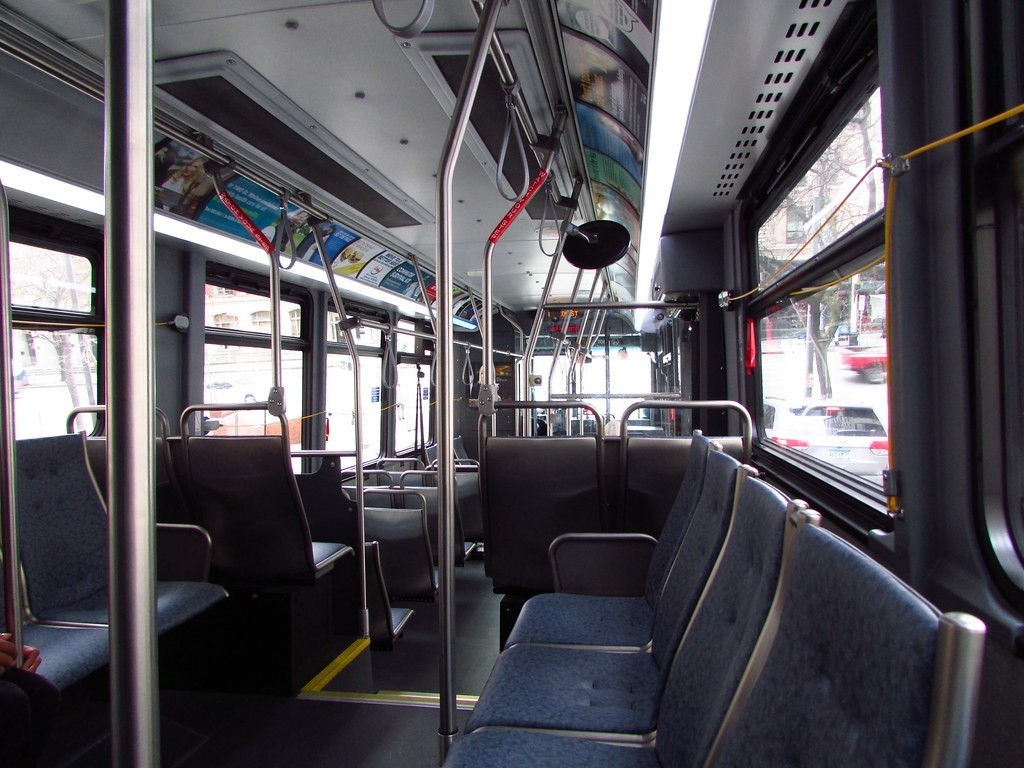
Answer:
[204,380,269,403]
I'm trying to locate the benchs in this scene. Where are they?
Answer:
[0,400,480,752]
[442,400,984,768]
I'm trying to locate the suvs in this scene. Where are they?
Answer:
[762,395,889,486]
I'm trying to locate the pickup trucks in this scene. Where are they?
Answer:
[839,344,888,385]
